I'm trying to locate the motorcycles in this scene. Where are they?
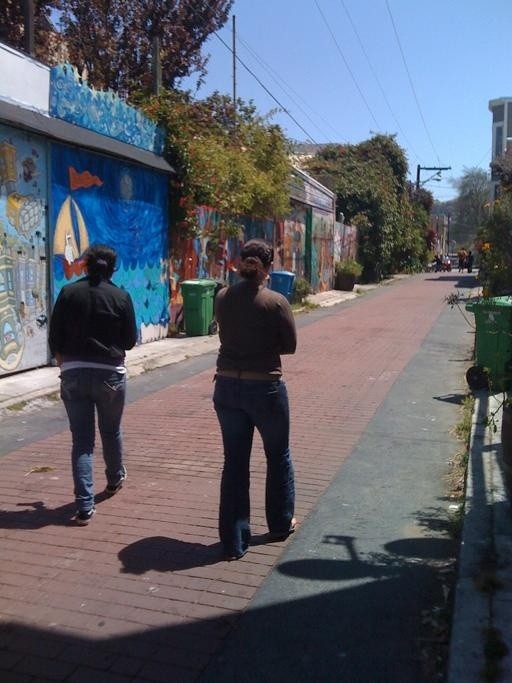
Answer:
[440,253,451,271]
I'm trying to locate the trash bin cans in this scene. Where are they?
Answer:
[179,278,217,336]
[466,296,512,393]
[269,271,296,305]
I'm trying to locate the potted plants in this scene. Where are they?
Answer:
[334,257,364,291]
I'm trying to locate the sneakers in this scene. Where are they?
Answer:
[76,505,96,526]
[289,517,297,532]
[105,465,127,494]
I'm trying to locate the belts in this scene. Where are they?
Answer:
[216,368,281,381]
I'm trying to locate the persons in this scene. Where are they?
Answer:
[212,236,299,562]
[433,247,474,274]
[48,243,137,524]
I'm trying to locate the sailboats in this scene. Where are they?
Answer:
[52,194,90,279]
